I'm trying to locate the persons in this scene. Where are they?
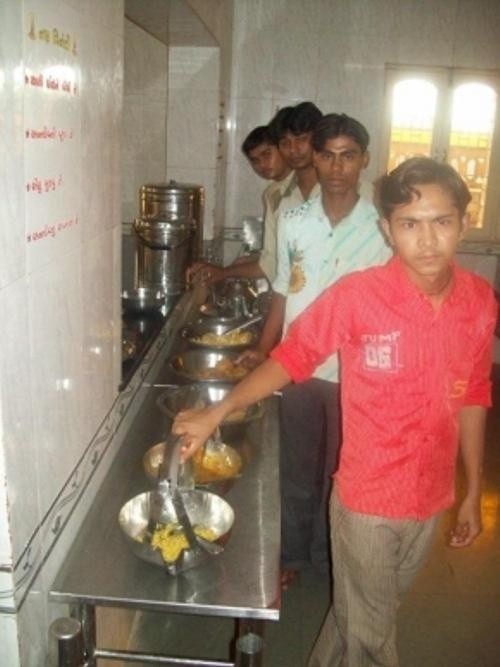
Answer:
[234,113,393,578]
[186,102,324,302]
[170,153,499,667]
[240,126,292,181]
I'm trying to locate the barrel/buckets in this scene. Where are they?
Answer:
[139,179,206,266]
[133,214,193,297]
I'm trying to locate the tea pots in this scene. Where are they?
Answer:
[214,274,273,320]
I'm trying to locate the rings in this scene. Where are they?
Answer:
[207,271,211,278]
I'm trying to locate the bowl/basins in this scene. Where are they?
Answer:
[178,316,267,351]
[121,285,168,315]
[155,380,267,439]
[142,437,245,493]
[115,486,234,578]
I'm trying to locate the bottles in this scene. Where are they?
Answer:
[160,347,262,391]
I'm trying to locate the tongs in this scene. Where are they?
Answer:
[132,429,207,558]
